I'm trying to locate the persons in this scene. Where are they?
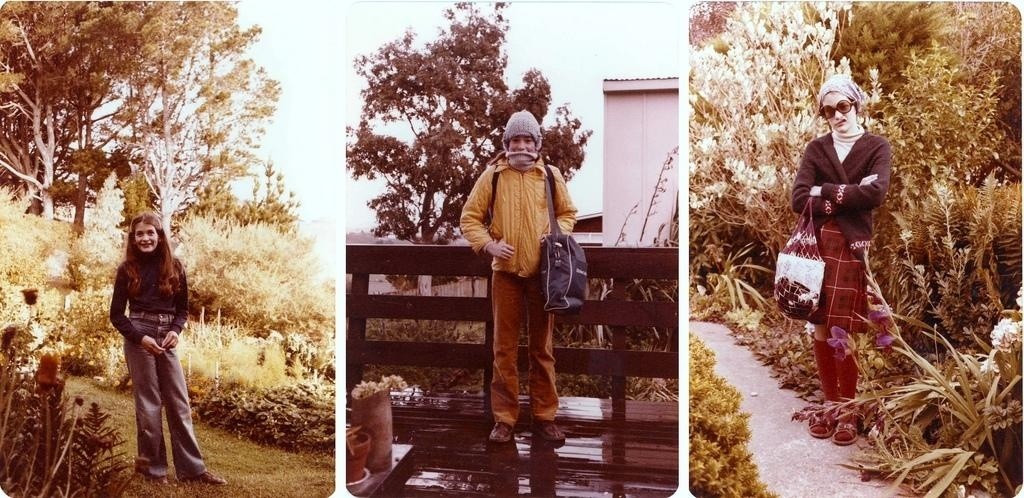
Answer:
[772,74,891,446]
[460,109,588,444]
[110,211,228,485]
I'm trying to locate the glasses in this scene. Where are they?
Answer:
[818,98,857,120]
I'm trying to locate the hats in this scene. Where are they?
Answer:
[502,110,543,153]
[817,75,863,105]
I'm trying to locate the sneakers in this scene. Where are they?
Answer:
[488,422,513,443]
[530,418,566,442]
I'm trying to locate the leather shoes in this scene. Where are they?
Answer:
[186,471,227,486]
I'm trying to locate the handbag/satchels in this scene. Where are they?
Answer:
[541,234,588,317]
[772,193,827,320]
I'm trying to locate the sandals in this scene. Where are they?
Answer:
[808,409,834,439]
[831,422,859,446]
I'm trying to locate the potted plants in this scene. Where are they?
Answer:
[346,379,396,485]
